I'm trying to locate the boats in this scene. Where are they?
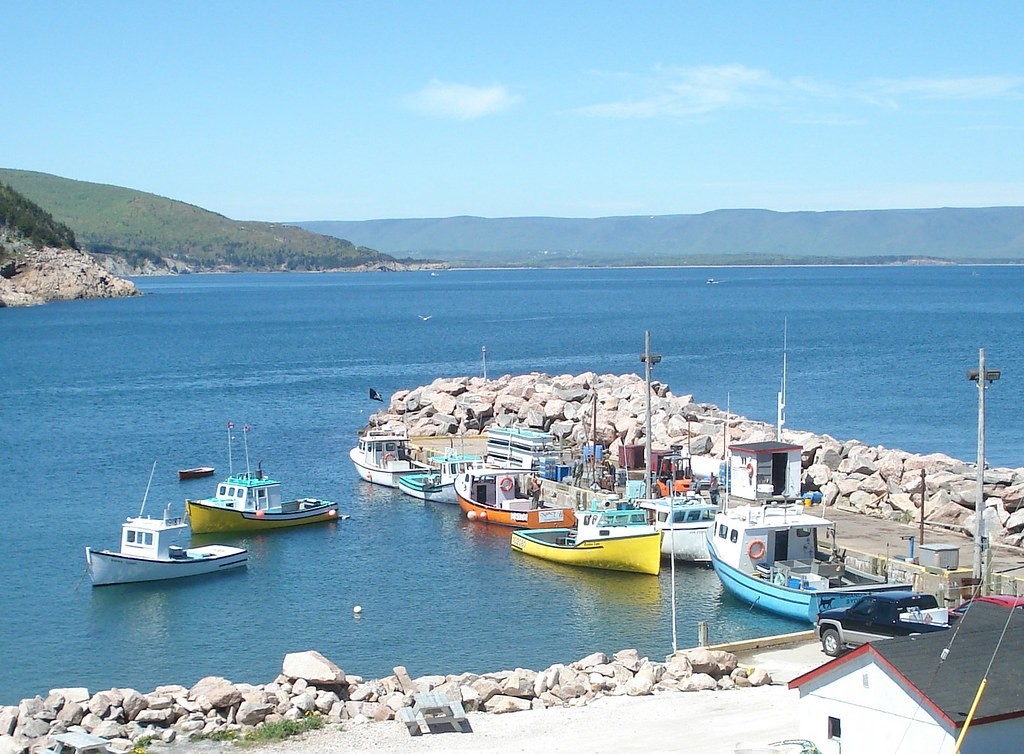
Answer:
[349,400,436,491]
[178,467,217,480]
[398,385,728,578]
[84,460,248,588]
[183,422,340,535]
[703,486,914,629]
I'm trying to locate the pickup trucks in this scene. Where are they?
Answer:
[813,589,955,658]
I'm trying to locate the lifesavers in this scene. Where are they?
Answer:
[748,540,765,559]
[384,453,397,465]
[500,478,513,492]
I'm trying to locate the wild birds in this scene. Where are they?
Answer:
[417,314,432,321]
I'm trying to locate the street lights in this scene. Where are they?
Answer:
[967,348,1001,601]
[641,329,661,524]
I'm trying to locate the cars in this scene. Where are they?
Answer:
[949,594,1024,628]
